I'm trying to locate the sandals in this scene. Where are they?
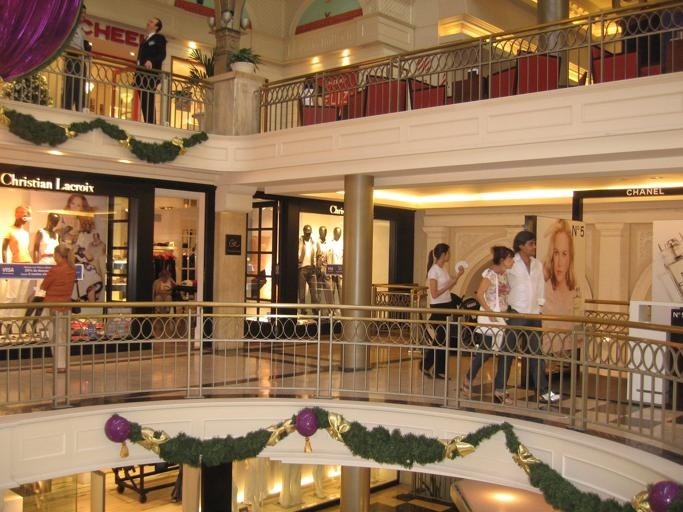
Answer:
[45,365,66,374]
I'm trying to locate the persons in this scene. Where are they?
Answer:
[297,224,344,323]
[57,194,107,314]
[0,205,33,335]
[154,270,178,338]
[419,242,465,380]
[481,231,560,401]
[39,244,77,372]
[539,220,581,353]
[246,257,255,283]
[131,17,166,124]
[60,4,93,112]
[462,244,515,402]
[300,79,323,106]
[20,213,61,336]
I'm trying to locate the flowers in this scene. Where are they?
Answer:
[0,72,54,106]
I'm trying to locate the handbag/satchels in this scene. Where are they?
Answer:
[475,309,508,336]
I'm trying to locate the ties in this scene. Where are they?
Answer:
[144,35,149,41]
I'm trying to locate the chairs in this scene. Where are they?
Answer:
[586,45,660,84]
[299,49,586,127]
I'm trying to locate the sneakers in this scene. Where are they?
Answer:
[417,362,560,406]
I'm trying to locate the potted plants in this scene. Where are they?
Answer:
[227,47,263,73]
